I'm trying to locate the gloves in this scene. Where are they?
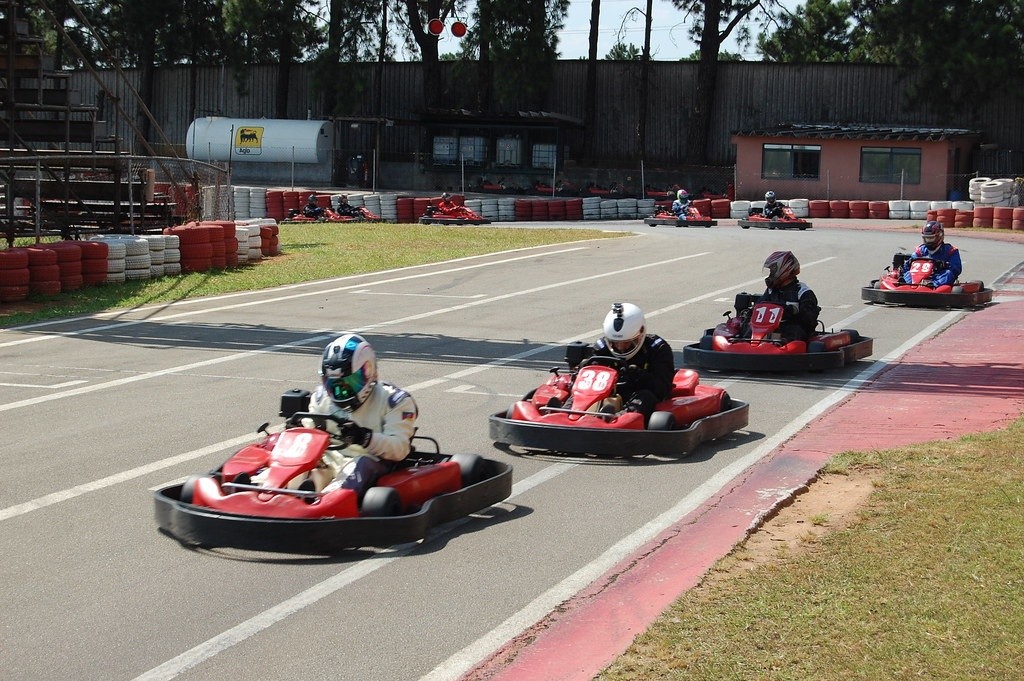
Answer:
[351,206,361,211]
[782,304,800,317]
[450,206,459,212]
[907,258,913,266]
[680,205,686,209]
[285,418,303,430]
[932,260,948,271]
[320,207,326,212]
[741,306,753,320]
[773,206,783,212]
[337,419,373,448]
[621,365,637,377]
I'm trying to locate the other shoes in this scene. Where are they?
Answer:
[543,396,563,415]
[784,216,787,219]
[360,215,365,220]
[594,403,615,424]
[730,335,747,343]
[232,472,251,493]
[295,479,316,505]
[925,281,937,291]
[897,277,906,286]
[686,212,691,215]
[462,216,468,220]
[772,338,787,346]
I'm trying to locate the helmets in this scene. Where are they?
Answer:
[441,193,453,203]
[322,333,379,413]
[602,302,647,361]
[921,221,944,250]
[308,194,319,206]
[676,189,688,204]
[765,191,776,205]
[337,195,348,205]
[763,251,801,290]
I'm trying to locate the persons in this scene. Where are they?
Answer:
[496,177,720,194]
[547,302,675,428]
[728,251,818,351]
[337,195,366,218]
[896,221,962,289]
[670,189,694,216]
[284,333,418,502]
[762,190,798,221]
[303,194,326,219]
[439,193,463,215]
